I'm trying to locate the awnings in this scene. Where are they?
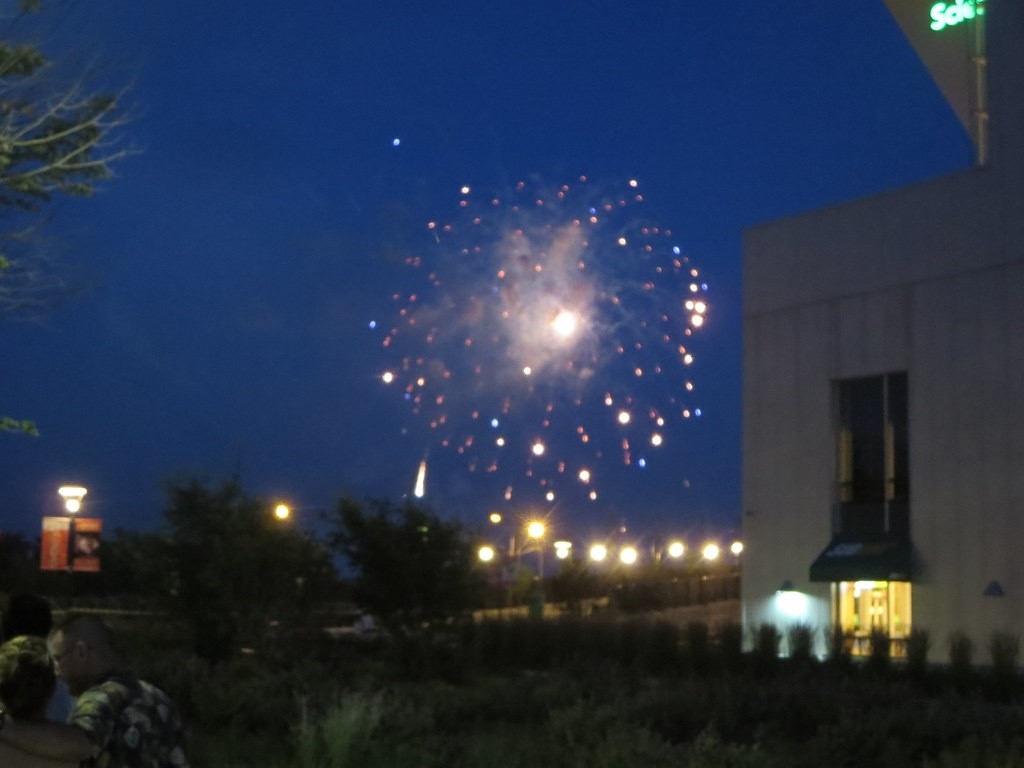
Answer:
[809,539,909,584]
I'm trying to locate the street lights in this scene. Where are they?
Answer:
[58,484,88,572]
[656,541,683,563]
[507,519,544,558]
[688,542,719,573]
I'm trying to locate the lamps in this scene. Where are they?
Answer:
[777,580,796,592]
[982,581,1004,597]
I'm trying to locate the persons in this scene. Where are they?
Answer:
[1,634,82,767]
[0,567,23,611]
[0,611,191,768]
[4,591,52,638]
